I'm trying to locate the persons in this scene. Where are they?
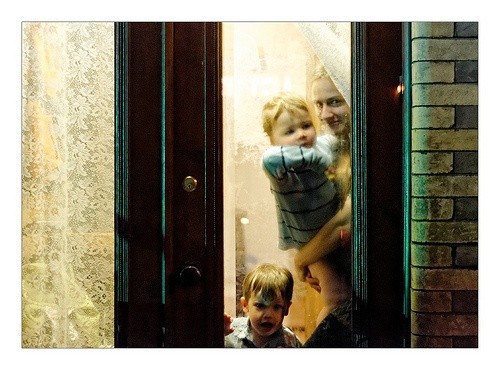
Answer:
[224,264,304,349]
[261,91,354,349]
[293,61,352,302]
